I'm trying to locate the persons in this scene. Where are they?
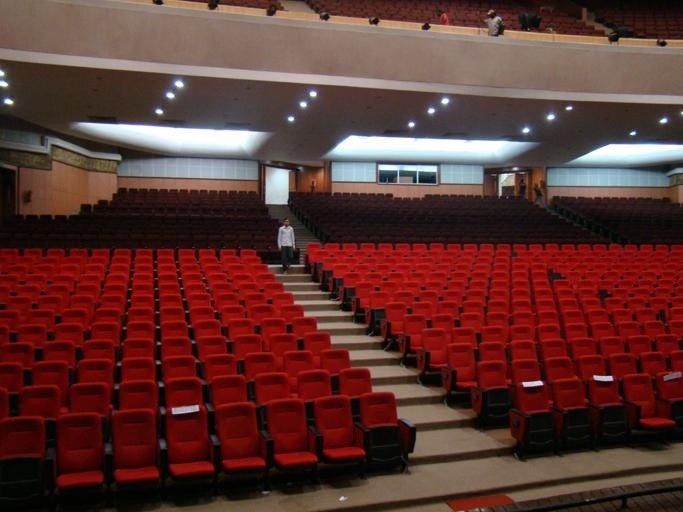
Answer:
[519,179,526,201]
[434,6,448,25]
[533,183,545,208]
[481,9,504,37]
[276,216,296,276]
